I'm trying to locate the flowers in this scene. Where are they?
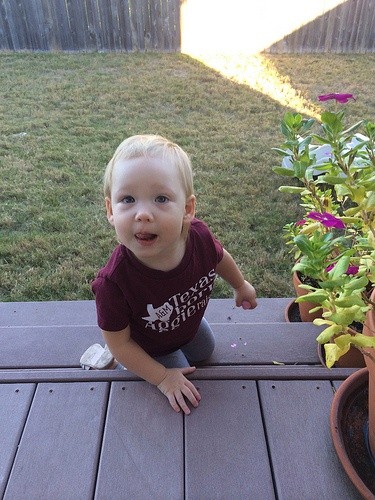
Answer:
[278,93,374,287]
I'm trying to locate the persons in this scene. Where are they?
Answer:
[79,132,259,417]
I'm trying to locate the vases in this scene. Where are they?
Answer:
[327,305,375,500]
[297,208,375,356]
[286,258,325,324]
[317,322,365,370]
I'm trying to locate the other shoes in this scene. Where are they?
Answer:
[79,342,117,370]
